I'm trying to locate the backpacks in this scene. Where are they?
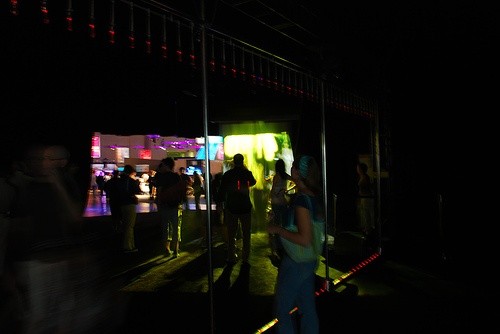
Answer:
[226,169,252,214]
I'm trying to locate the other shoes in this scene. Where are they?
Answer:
[243,262,249,266]
[122,248,138,253]
[166,248,179,257]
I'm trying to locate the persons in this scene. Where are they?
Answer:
[0,146,81,334]
[356,163,375,228]
[271,160,292,240]
[267,156,326,334]
[217,154,256,264]
[95,158,223,255]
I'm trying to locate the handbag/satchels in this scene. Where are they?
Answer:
[280,193,325,263]
